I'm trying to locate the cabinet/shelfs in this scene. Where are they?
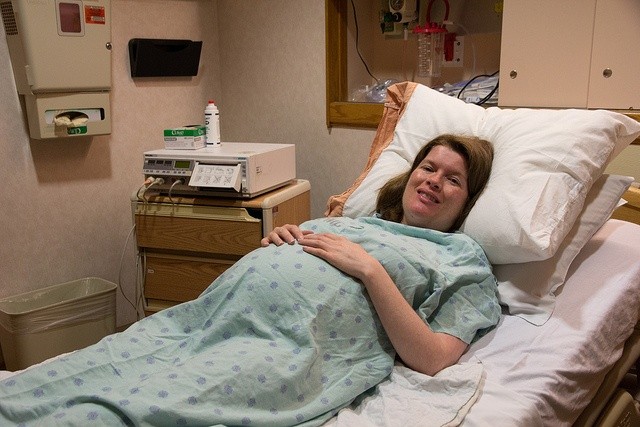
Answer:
[129,177,312,318]
[497,1,639,110]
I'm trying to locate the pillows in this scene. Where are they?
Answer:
[494,176,635,327]
[322,79,639,266]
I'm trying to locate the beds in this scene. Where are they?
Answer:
[1,190,639,427]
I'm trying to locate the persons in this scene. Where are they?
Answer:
[0,132,504,427]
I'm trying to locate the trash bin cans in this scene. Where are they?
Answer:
[0,277,118,373]
